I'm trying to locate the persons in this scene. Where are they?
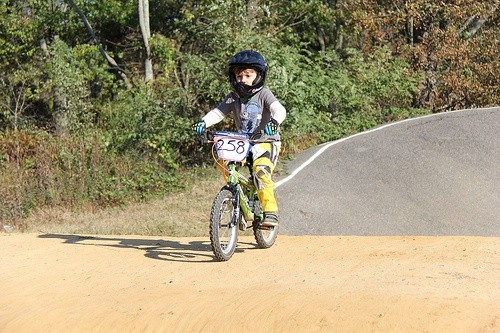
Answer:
[191,48,286,227]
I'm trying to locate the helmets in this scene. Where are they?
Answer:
[228,49,268,98]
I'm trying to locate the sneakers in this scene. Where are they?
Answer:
[261,212,279,226]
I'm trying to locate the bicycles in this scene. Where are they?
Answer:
[186,120,279,261]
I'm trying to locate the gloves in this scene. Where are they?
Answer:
[192,119,206,136]
[264,117,279,136]
